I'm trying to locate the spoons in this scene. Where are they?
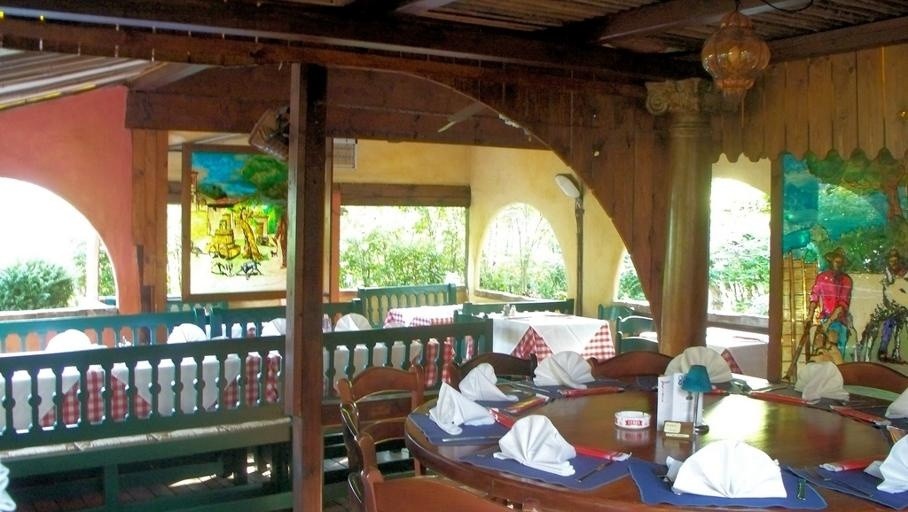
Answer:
[651,464,683,496]
[805,465,875,498]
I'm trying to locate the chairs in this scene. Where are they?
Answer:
[587,304,675,354]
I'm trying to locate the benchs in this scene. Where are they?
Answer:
[1,312,492,506]
[208,298,364,342]
[359,281,612,377]
[1,305,208,354]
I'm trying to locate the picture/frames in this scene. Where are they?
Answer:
[180,140,289,303]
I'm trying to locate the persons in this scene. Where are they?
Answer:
[807,248,854,355]
[877,249,908,364]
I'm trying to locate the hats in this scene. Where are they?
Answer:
[824,246,852,267]
[882,248,903,259]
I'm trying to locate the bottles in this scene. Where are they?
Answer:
[322,313,333,333]
[193,304,213,324]
[502,304,517,317]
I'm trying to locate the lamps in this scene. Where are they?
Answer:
[245,105,290,162]
[553,174,581,199]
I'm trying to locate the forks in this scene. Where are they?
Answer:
[576,448,629,481]
